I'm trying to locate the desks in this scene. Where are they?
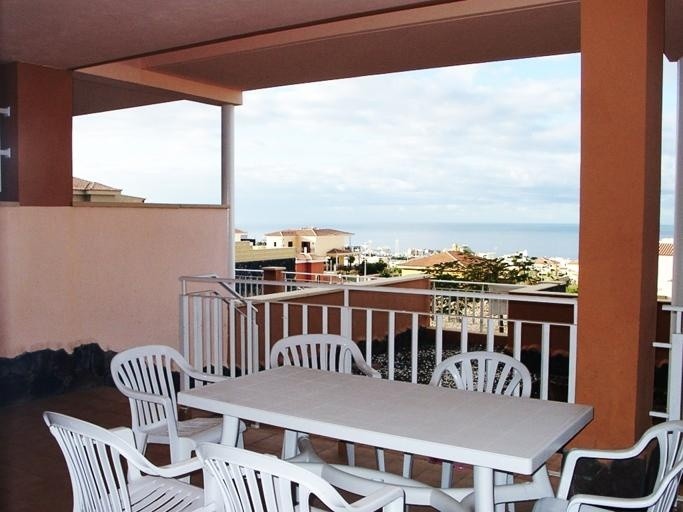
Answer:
[178,362,595,512]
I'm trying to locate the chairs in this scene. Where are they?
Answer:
[42,411,205,512]
[109,343,248,485]
[529,419,683,512]
[268,332,386,474]
[190,439,406,512]
[402,350,532,511]
